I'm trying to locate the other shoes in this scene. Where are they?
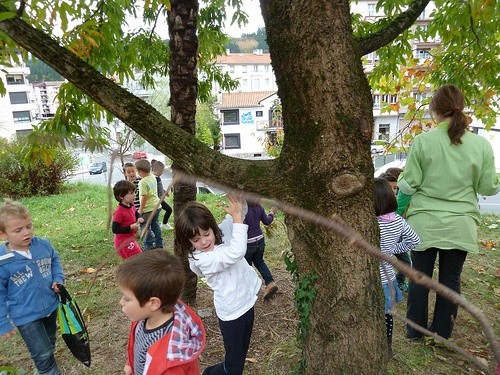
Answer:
[263,282,278,299]
[397,282,408,292]
[428,322,432,328]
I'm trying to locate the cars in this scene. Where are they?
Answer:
[370,140,390,154]
[89,161,107,175]
[132,149,147,159]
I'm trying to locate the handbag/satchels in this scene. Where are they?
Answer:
[55,284,91,368]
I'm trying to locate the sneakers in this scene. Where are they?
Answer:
[161,222,174,230]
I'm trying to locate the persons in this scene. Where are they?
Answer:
[151,159,174,230]
[135,160,163,252]
[0,198,65,375]
[123,163,145,239]
[112,180,144,261]
[244,200,279,300]
[175,190,262,375]
[394,84,500,340]
[372,167,421,362]
[116,247,205,375]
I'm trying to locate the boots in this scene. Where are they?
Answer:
[387,336,393,359]
